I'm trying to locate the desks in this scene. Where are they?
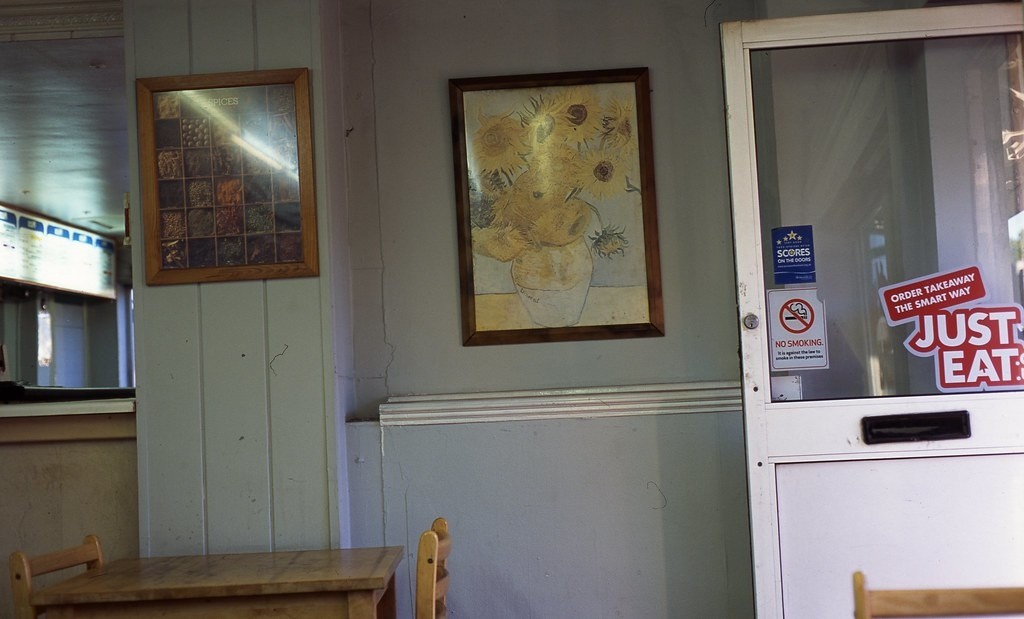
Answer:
[27,544,407,619]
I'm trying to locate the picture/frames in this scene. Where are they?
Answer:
[133,66,322,286]
[448,66,667,349]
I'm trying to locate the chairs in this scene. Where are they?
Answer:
[7,535,104,619]
[415,518,451,619]
[852,570,1024,619]
